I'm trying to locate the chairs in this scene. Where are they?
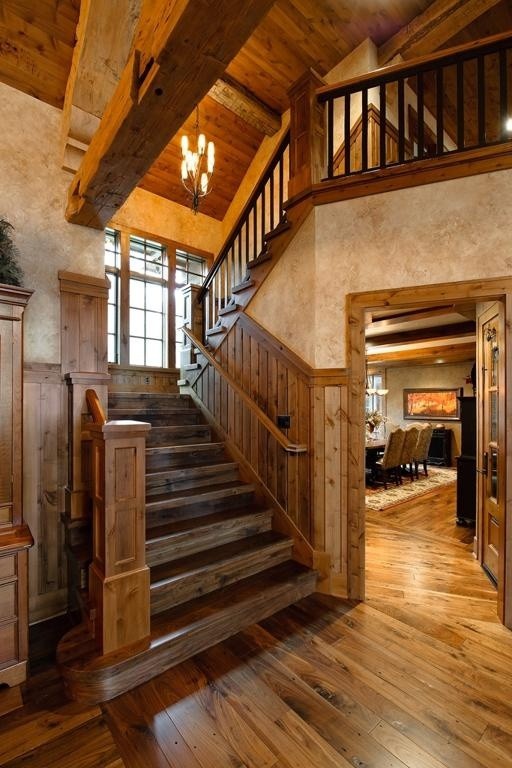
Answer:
[376,422,434,490]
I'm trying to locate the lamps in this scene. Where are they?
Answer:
[180,106,216,217]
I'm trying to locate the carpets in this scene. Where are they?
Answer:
[365,463,460,511]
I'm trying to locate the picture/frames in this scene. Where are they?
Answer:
[402,387,462,420]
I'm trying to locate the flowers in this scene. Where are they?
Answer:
[366,412,389,430]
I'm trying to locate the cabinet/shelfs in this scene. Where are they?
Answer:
[454,396,479,529]
